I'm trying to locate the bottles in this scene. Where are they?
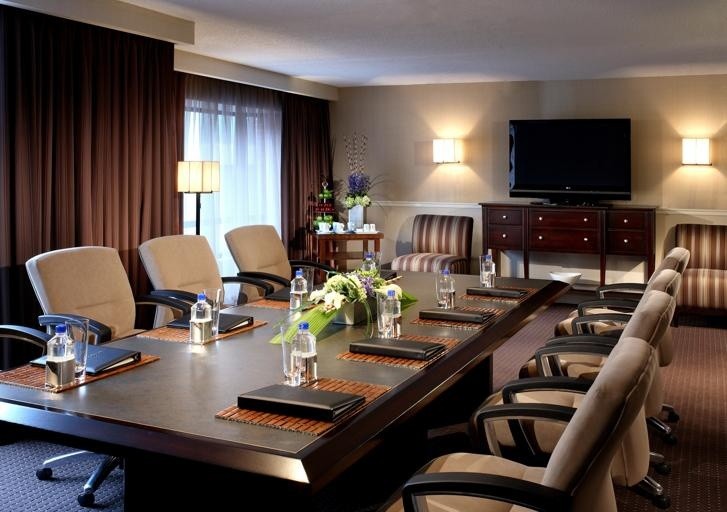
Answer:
[293,322,319,383]
[45,324,75,387]
[386,291,402,337]
[442,271,455,306]
[483,255,496,287]
[290,270,306,310]
[190,293,212,342]
[360,252,375,275]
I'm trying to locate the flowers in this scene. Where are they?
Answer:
[343,130,372,209]
[266,269,418,344]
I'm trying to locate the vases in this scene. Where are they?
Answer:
[331,300,377,326]
[348,205,364,228]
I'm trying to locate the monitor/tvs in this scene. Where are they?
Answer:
[509,118,631,208]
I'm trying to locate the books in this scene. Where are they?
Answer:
[238,383,366,424]
[419,308,495,323]
[29,342,141,376]
[166,312,253,334]
[264,287,291,302]
[466,287,527,298]
[350,338,446,361]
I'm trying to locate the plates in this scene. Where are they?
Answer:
[315,230,333,234]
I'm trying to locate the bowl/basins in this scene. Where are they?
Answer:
[549,271,582,286]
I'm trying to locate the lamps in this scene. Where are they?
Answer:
[432,139,460,164]
[176,161,220,235]
[681,137,712,166]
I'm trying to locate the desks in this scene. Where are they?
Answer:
[0,269,570,512]
[313,232,384,283]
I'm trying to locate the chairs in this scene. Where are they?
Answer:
[0,322,53,373]
[138,235,274,329]
[546,267,683,455]
[224,224,336,306]
[391,215,474,274]
[379,335,661,512]
[555,248,690,379]
[472,286,678,501]
[673,223,727,327]
[24,245,193,507]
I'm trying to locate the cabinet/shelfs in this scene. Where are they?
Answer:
[478,203,656,287]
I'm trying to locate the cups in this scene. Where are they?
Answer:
[372,253,382,278]
[333,222,379,235]
[300,266,314,301]
[479,255,492,288]
[436,273,452,309]
[279,325,301,385]
[376,290,395,339]
[319,222,331,232]
[204,288,220,336]
[65,319,88,382]
